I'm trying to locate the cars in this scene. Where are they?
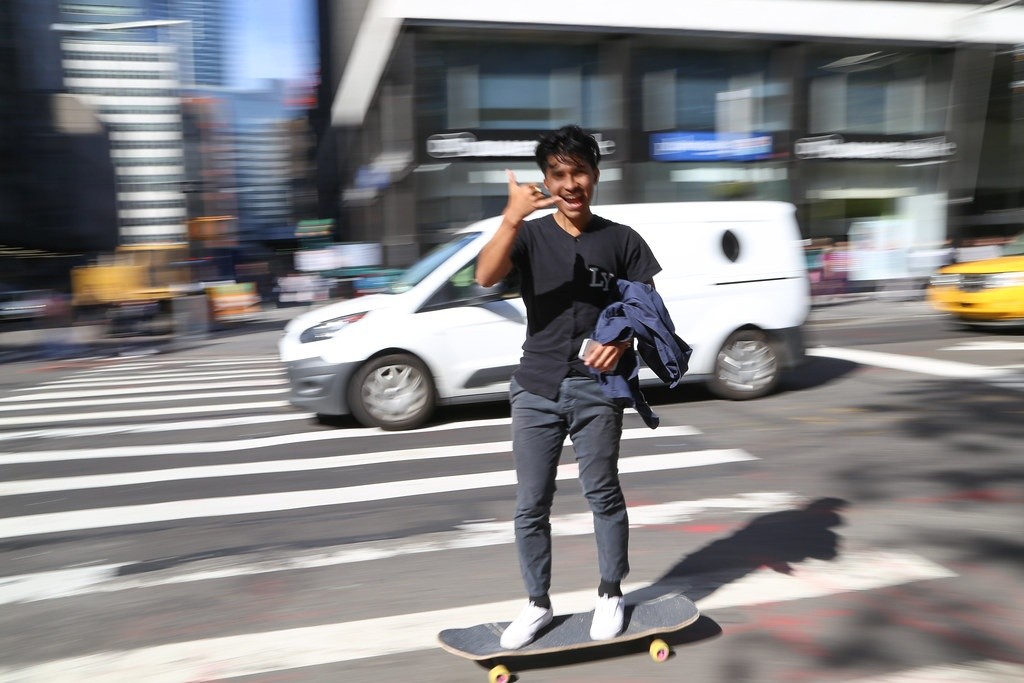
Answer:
[922,235,1024,327]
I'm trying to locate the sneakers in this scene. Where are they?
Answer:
[499,605,553,649]
[590,595,626,640]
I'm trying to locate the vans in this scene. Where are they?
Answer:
[280,198,821,430]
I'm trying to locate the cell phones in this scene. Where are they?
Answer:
[578,339,618,372]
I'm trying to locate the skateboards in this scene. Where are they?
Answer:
[436,594,701,683]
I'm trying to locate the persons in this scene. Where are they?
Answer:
[475,121,665,648]
